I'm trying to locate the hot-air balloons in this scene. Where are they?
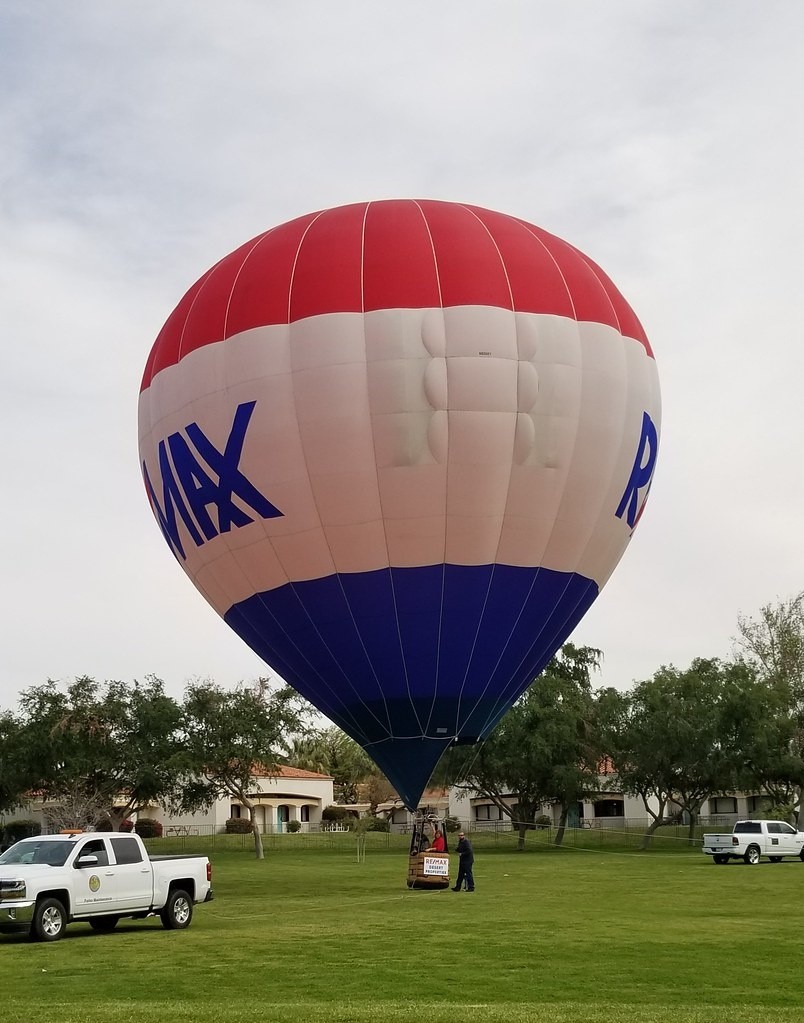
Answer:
[139,197,657,893]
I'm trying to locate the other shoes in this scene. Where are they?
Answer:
[451,887,462,892]
[466,887,474,893]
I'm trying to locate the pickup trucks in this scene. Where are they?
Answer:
[0,831,214,944]
[701,819,804,866]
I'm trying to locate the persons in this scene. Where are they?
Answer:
[451,831,475,891]
[418,834,431,852]
[425,830,445,852]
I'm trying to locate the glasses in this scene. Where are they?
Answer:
[458,835,464,836]
